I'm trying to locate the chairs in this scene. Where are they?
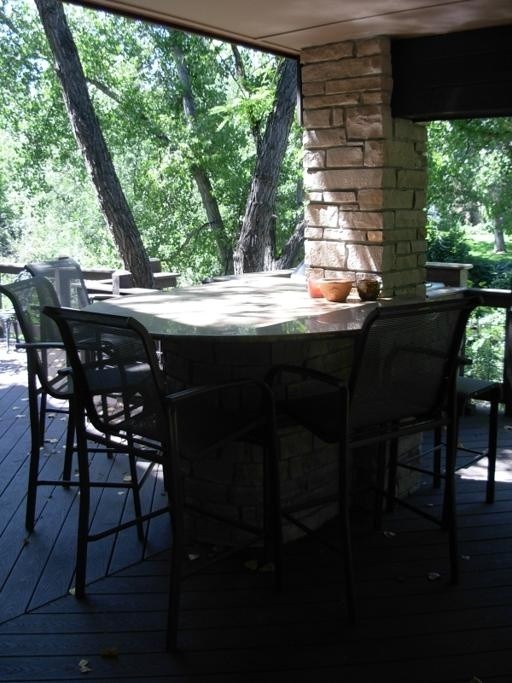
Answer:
[1,254,510,653]
